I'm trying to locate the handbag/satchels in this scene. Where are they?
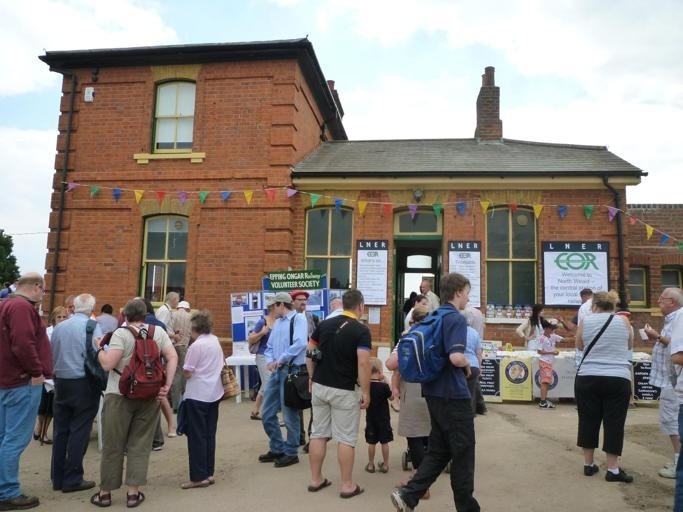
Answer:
[85,320,107,391]
[284,372,311,410]
[177,393,185,436]
[221,360,241,400]
[249,319,267,353]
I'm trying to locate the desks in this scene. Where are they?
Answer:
[529,348,635,406]
[225,356,258,404]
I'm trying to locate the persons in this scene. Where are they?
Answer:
[642,287,681,480]
[532,315,570,410]
[390,272,481,512]
[0,272,194,512]
[247,289,392,498]
[572,288,634,484]
[384,279,486,499]
[181,309,223,489]
[515,288,595,370]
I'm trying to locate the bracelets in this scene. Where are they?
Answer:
[655,335,663,342]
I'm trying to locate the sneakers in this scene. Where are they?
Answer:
[391,489,414,511]
[657,467,676,478]
[584,464,599,476]
[539,400,555,408]
[664,463,674,469]
[605,467,633,483]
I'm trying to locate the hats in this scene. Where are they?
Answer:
[176,301,191,312]
[539,317,558,329]
[267,292,293,306]
[289,290,310,298]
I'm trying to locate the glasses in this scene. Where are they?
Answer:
[659,296,670,303]
[36,283,46,294]
[297,298,307,301]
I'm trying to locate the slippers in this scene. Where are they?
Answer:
[340,483,364,498]
[308,479,332,491]
[180,480,210,489]
[365,463,375,473]
[378,461,388,473]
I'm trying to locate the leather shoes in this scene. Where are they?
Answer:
[0,495,39,509]
[33,432,41,440]
[168,431,176,437]
[259,440,309,467]
[62,479,95,493]
[43,439,54,444]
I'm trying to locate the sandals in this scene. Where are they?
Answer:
[250,412,262,420]
[91,492,112,507]
[126,491,145,507]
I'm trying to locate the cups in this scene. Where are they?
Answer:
[505,342,512,353]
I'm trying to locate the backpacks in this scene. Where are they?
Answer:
[119,325,167,400]
[397,307,459,384]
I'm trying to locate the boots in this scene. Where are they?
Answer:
[208,476,215,484]
